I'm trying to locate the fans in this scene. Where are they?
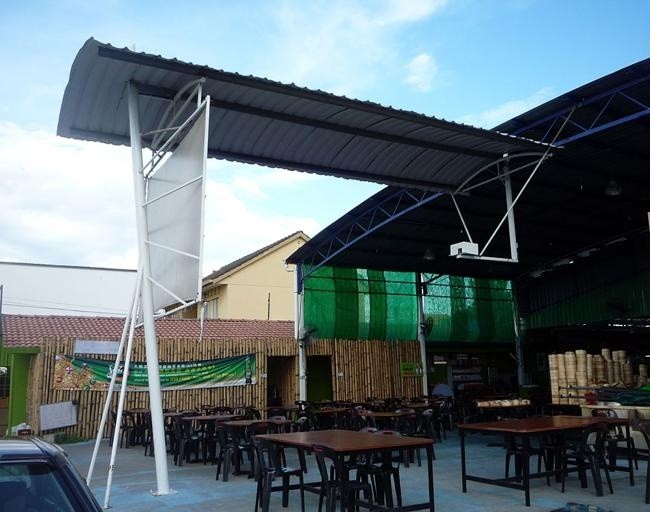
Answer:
[419,317,433,336]
[298,323,321,349]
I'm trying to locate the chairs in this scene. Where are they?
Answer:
[360,430,403,506]
[466,394,650,504]
[251,437,304,510]
[311,443,373,510]
[331,427,376,499]
[110,395,454,481]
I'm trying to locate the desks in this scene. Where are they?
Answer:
[255,429,434,511]
[458,415,632,507]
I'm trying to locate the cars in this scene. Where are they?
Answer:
[0,436,102,512]
